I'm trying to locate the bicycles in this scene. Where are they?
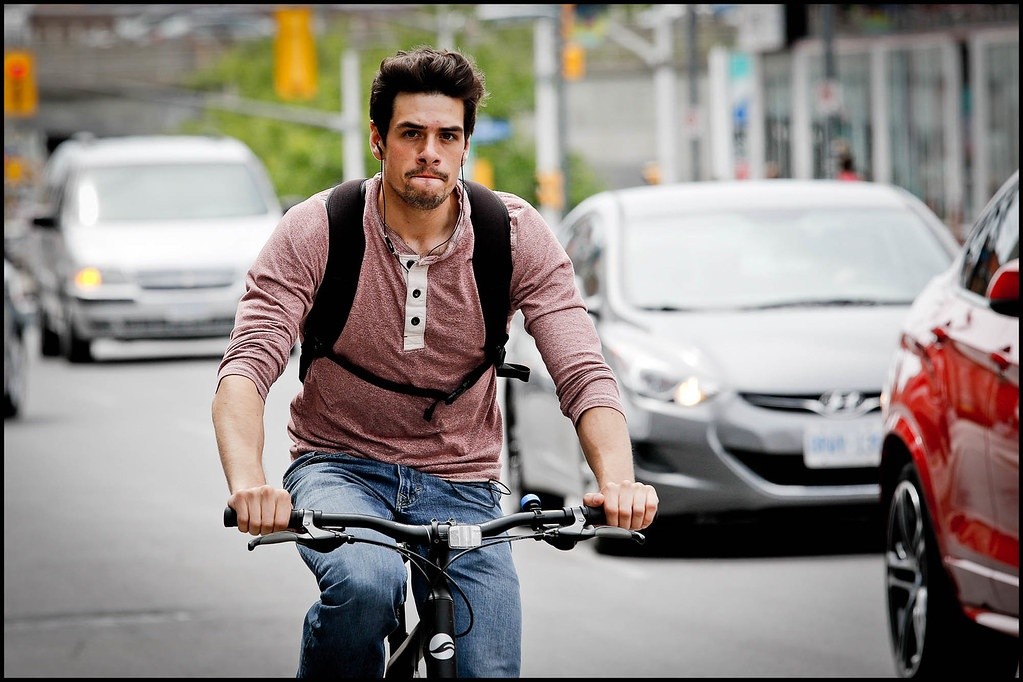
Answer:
[223,491,659,682]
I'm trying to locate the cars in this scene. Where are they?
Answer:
[877,169,1023,682]
[499,175,963,536]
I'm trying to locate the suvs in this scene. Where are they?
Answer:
[6,127,290,364]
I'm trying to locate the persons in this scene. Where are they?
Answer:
[212,46,660,677]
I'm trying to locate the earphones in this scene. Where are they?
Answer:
[377,140,382,156]
[461,150,466,164]
